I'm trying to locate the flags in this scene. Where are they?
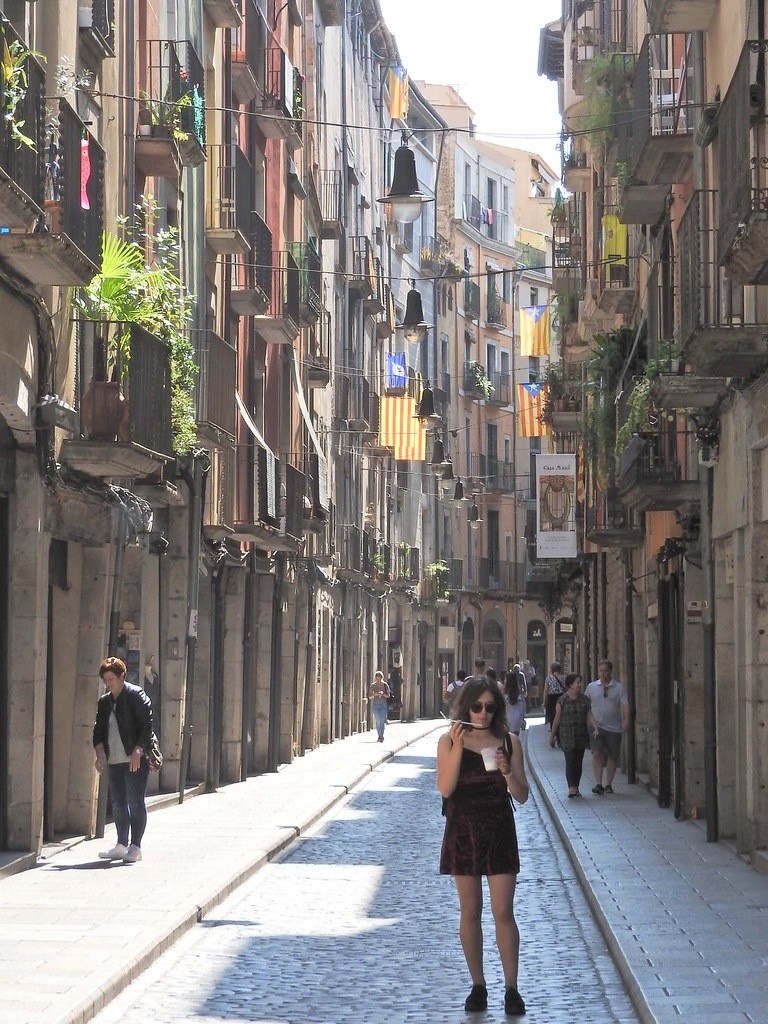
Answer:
[520,304,551,356]
[378,395,417,446]
[395,418,428,460]
[389,66,409,119]
[516,382,554,438]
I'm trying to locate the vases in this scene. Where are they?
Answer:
[41,200,67,233]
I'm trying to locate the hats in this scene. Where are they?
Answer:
[524,660,530,664]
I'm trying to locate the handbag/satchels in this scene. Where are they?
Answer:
[145,749,163,773]
[384,683,394,706]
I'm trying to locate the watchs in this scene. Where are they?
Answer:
[133,748,142,754]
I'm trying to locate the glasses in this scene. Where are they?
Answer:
[604,687,608,697]
[471,702,497,713]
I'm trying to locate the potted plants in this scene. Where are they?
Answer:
[70,233,179,439]
[139,88,194,140]
[255,80,281,110]
[544,387,579,412]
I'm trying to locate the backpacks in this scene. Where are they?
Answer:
[449,682,465,707]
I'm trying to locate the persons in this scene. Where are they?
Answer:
[437,673,530,1016]
[367,671,390,742]
[548,672,598,798]
[442,656,572,748]
[91,656,164,863]
[581,660,630,795]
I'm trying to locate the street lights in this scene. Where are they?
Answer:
[687,401,721,843]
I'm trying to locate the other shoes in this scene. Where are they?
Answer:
[568,794,574,798]
[465,986,487,1010]
[505,988,525,1014]
[123,844,142,862]
[574,791,581,797]
[377,738,379,742]
[380,737,384,742]
[592,786,604,795]
[605,785,613,792]
[99,843,129,858]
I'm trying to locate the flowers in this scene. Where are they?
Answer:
[44,55,95,201]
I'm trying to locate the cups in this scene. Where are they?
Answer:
[480,747,499,772]
